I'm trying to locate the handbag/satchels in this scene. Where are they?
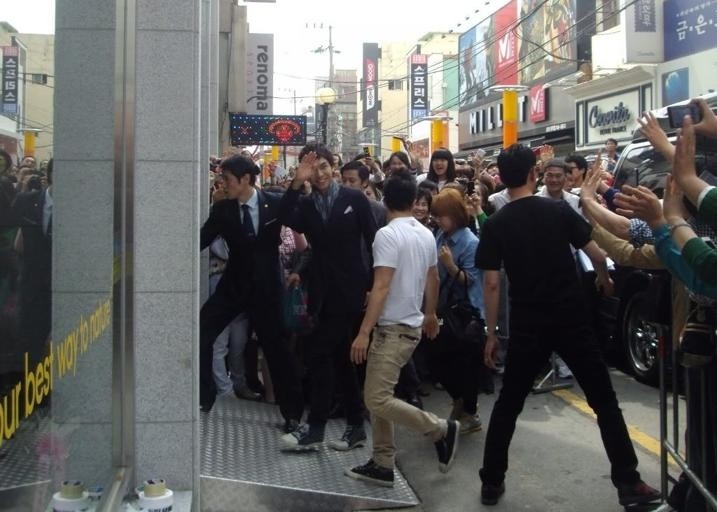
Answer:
[443,268,486,372]
[679,306,717,356]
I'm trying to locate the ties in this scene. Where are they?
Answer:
[241,204,255,240]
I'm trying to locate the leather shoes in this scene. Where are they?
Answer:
[435,382,446,391]
[482,481,505,504]
[407,393,423,410]
[418,387,430,397]
[618,479,661,505]
[284,419,299,433]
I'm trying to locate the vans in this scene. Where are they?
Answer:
[579,87,716,387]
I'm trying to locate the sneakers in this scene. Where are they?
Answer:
[459,413,482,435]
[333,424,367,451]
[279,422,326,452]
[556,357,574,379]
[344,459,395,487]
[449,412,481,422]
[236,385,263,401]
[434,420,460,474]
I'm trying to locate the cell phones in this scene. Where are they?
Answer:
[467,182,474,196]
[631,168,638,189]
[365,154,370,165]
[474,149,486,162]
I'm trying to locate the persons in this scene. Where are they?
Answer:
[0,148,52,439]
[199,98,717,510]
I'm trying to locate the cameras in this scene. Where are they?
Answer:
[667,103,703,129]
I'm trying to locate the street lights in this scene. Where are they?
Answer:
[356,143,379,155]
[257,159,264,186]
[489,83,530,151]
[314,87,336,147]
[14,124,45,163]
[272,144,280,166]
[383,133,408,155]
[420,116,453,154]
